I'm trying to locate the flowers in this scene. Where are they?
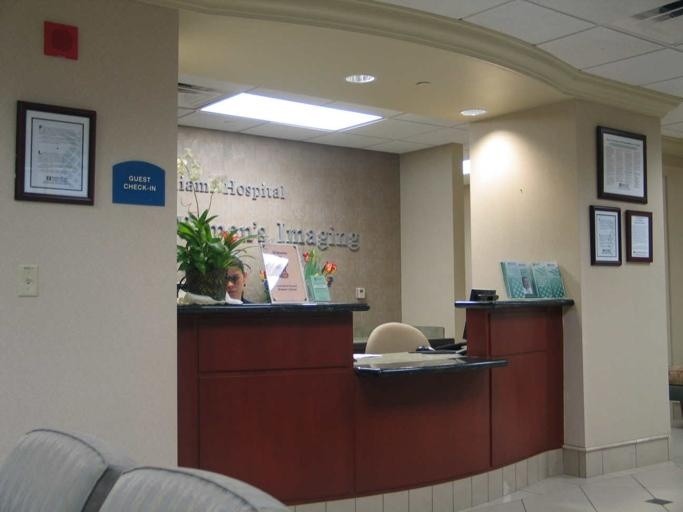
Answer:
[260,270,271,290]
[177,145,257,279]
[302,248,337,288]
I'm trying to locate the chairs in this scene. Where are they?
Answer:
[364,322,433,355]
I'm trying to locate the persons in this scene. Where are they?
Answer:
[226,257,255,304]
[521,276,534,295]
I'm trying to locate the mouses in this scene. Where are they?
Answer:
[416,345,435,351]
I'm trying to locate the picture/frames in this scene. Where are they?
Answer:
[625,208,653,263]
[589,206,622,267]
[15,99,97,203]
[595,125,647,202]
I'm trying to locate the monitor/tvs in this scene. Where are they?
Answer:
[460,288,498,357]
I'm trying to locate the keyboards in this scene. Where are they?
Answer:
[436,341,467,354]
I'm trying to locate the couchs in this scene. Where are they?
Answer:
[0,424,292,511]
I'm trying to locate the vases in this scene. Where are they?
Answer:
[183,265,227,303]
[265,290,272,304]
